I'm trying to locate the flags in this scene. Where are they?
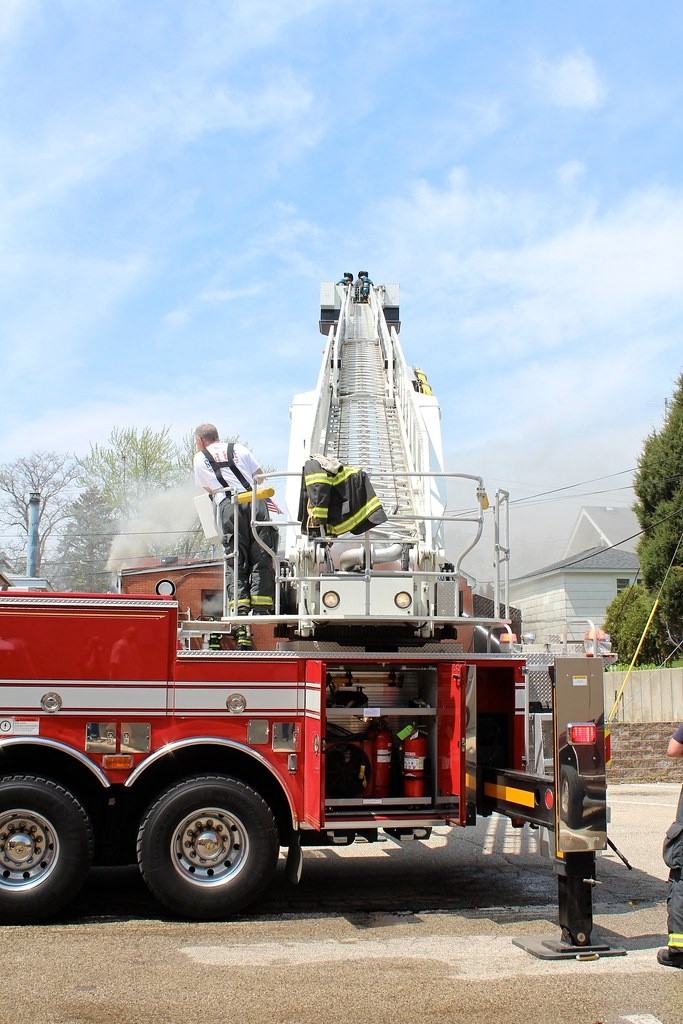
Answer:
[266,498,284,515]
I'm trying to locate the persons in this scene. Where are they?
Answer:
[657,723,683,969]
[336,272,354,286]
[193,422,281,617]
[353,271,374,303]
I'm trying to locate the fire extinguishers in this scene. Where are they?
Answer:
[400,722,428,796]
[371,717,394,799]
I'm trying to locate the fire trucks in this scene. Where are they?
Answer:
[0,282,617,923]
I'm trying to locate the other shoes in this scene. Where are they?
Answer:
[657,947,683,969]
[237,606,249,615]
[253,610,271,615]
